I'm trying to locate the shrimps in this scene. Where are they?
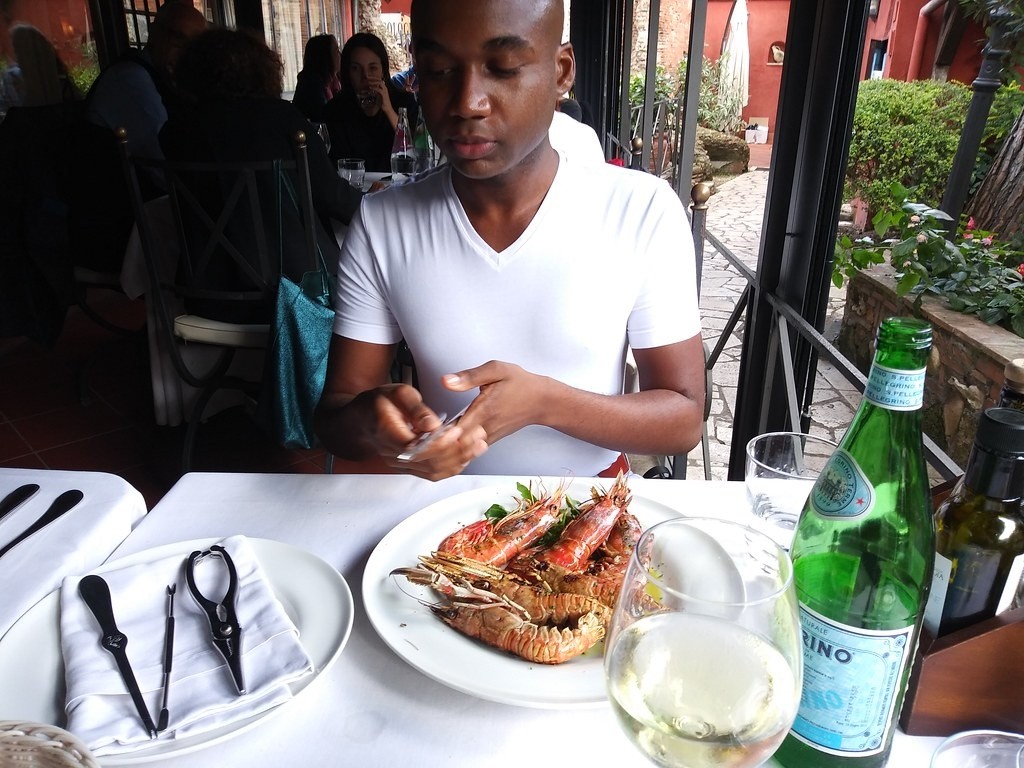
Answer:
[388,468,674,665]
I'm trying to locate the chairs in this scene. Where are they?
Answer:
[115,125,324,473]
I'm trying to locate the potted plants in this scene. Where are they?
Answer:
[617,64,673,174]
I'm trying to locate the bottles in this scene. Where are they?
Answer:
[922,356,1024,637]
[412,105,435,173]
[390,108,416,179]
[771,313,938,766]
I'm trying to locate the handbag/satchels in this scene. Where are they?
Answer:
[274,159,339,448]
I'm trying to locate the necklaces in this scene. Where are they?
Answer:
[359,96,372,103]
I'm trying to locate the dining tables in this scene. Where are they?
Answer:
[0,466,1024,768]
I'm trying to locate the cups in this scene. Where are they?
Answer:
[602,513,807,768]
[930,729,1024,768]
[360,77,381,110]
[338,159,365,191]
[744,429,851,533]
[310,123,331,155]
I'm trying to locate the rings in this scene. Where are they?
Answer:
[382,81,385,84]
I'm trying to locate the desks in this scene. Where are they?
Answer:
[118,171,419,429]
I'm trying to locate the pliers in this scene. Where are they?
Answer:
[185,541,247,699]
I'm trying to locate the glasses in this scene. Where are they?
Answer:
[157,20,189,48]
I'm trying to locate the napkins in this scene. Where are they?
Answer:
[59,535,314,756]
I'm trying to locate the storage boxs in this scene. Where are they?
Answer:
[739,117,769,144]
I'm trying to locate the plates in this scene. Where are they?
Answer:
[0,532,356,767]
[359,479,753,713]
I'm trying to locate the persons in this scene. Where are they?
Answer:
[319,33,418,173]
[555,81,582,123]
[10,28,79,106]
[292,34,341,117]
[158,28,390,324]
[390,43,421,106]
[315,0,705,482]
[84,3,207,309]
